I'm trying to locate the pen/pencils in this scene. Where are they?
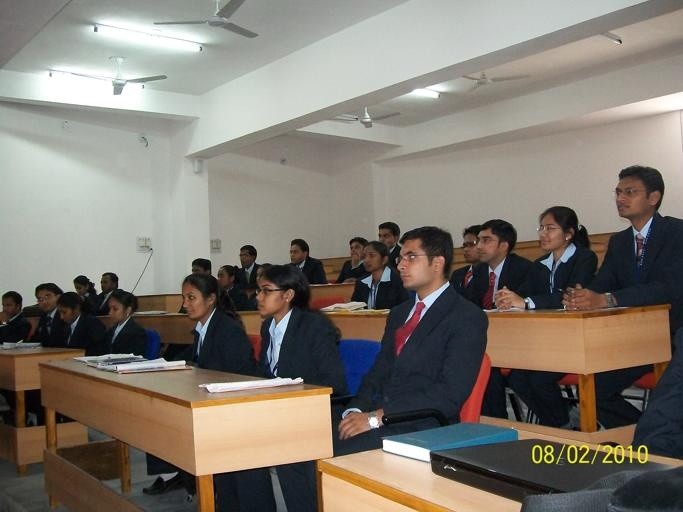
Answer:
[17,339,23,344]
[558,288,572,295]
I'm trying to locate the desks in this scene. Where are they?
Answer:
[317,414,683,512]
[319,239,623,283]
[87,310,265,361]
[1,347,86,478]
[37,355,336,512]
[304,281,366,321]
[320,304,672,450]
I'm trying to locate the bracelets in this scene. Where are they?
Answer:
[604,290,616,309]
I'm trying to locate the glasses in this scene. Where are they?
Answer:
[614,189,644,194]
[256,288,283,295]
[474,239,496,246]
[396,255,432,264]
[537,226,560,231]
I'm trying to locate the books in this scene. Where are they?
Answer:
[76,351,193,376]
[379,419,518,463]
[319,301,366,312]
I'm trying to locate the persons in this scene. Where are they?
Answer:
[211,265,342,511]
[213,264,248,311]
[560,165,680,424]
[631,307,680,459]
[242,263,273,311]
[234,245,260,285]
[493,204,598,429]
[350,241,408,311]
[464,217,534,420]
[289,239,329,284]
[274,226,490,511]
[177,258,211,312]
[0,272,146,428]
[449,225,484,303]
[179,272,257,376]
[376,221,403,264]
[335,236,367,284]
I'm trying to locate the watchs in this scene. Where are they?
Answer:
[522,298,531,309]
[365,407,382,430]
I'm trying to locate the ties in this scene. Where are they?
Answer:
[395,302,425,356]
[482,272,496,309]
[636,234,644,269]
[463,272,472,288]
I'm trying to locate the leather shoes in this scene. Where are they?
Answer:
[143,474,183,494]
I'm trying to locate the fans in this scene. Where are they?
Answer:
[327,107,401,129]
[154,0,259,40]
[454,71,528,94]
[71,58,166,96]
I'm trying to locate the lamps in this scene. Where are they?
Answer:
[50,70,144,90]
[94,23,203,53]
[600,31,622,46]
[412,87,443,101]
